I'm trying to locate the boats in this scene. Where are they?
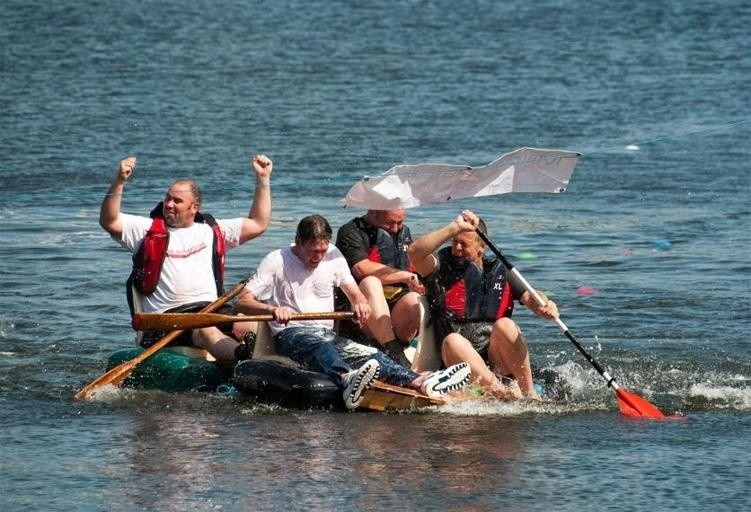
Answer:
[105,347,575,413]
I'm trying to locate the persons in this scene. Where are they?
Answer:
[236,213,471,409]
[407,210,558,404]
[98,154,272,361]
[333,210,431,372]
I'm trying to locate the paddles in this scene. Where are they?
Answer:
[475,226,666,418]
[74,279,250,400]
[132,311,358,330]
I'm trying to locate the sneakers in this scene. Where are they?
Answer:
[234,330,257,361]
[418,362,471,397]
[340,358,380,409]
